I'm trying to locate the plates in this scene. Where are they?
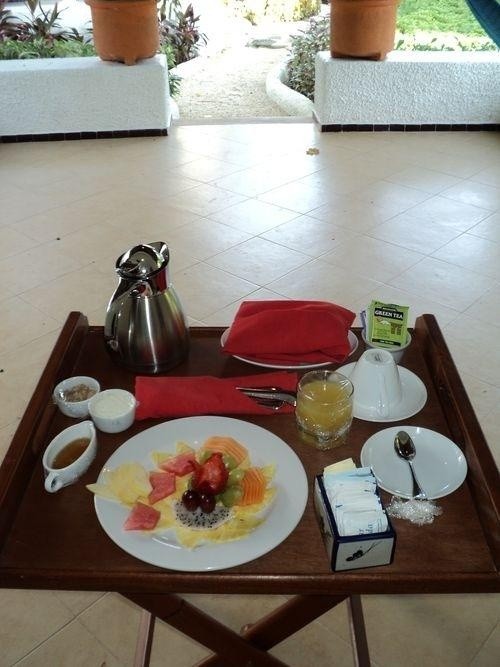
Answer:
[358,426,469,499]
[220,327,359,368]
[326,362,428,425]
[92,414,310,575]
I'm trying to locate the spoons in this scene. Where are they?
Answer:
[393,430,428,502]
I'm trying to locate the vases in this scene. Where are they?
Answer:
[329,2,400,62]
[85,0,161,66]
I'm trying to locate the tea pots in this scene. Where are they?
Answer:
[102,240,191,374]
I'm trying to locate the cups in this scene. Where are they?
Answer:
[42,419,96,495]
[295,369,354,451]
[348,348,403,418]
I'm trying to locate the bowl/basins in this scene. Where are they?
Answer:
[54,375,100,417]
[361,326,412,363]
[88,387,138,434]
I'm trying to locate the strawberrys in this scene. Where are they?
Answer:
[192,450,226,496]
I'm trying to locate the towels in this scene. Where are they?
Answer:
[133,370,300,419]
[221,299,357,365]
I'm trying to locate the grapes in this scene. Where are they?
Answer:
[185,450,246,508]
[183,488,197,512]
[199,492,214,513]
